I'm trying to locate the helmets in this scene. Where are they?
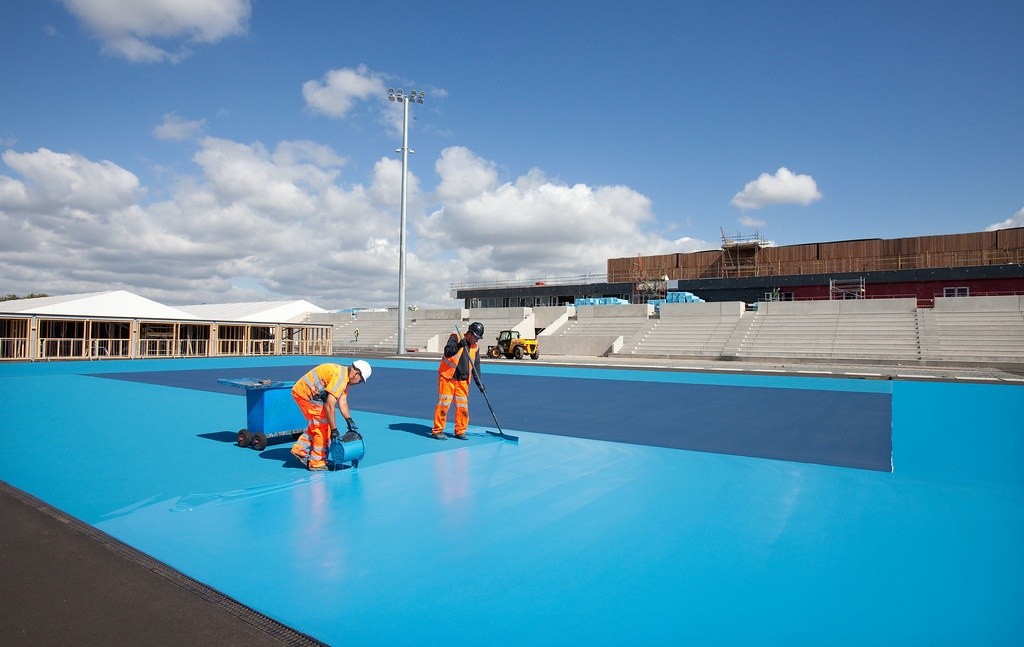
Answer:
[352,360,372,383]
[469,322,484,339]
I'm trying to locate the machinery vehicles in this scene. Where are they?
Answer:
[487,330,539,360]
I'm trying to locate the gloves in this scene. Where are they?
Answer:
[476,381,486,393]
[330,428,339,441]
[459,340,466,347]
[346,418,358,431]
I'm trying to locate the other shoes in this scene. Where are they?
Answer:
[309,465,334,471]
[291,451,310,466]
[432,431,448,440]
[455,434,468,440]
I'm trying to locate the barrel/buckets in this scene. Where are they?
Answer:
[330,430,365,464]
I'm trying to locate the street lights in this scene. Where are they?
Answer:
[387,88,426,356]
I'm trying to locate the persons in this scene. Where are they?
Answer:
[290,359,372,470]
[432,322,484,440]
[408,305,418,311]
[354,329,360,341]
